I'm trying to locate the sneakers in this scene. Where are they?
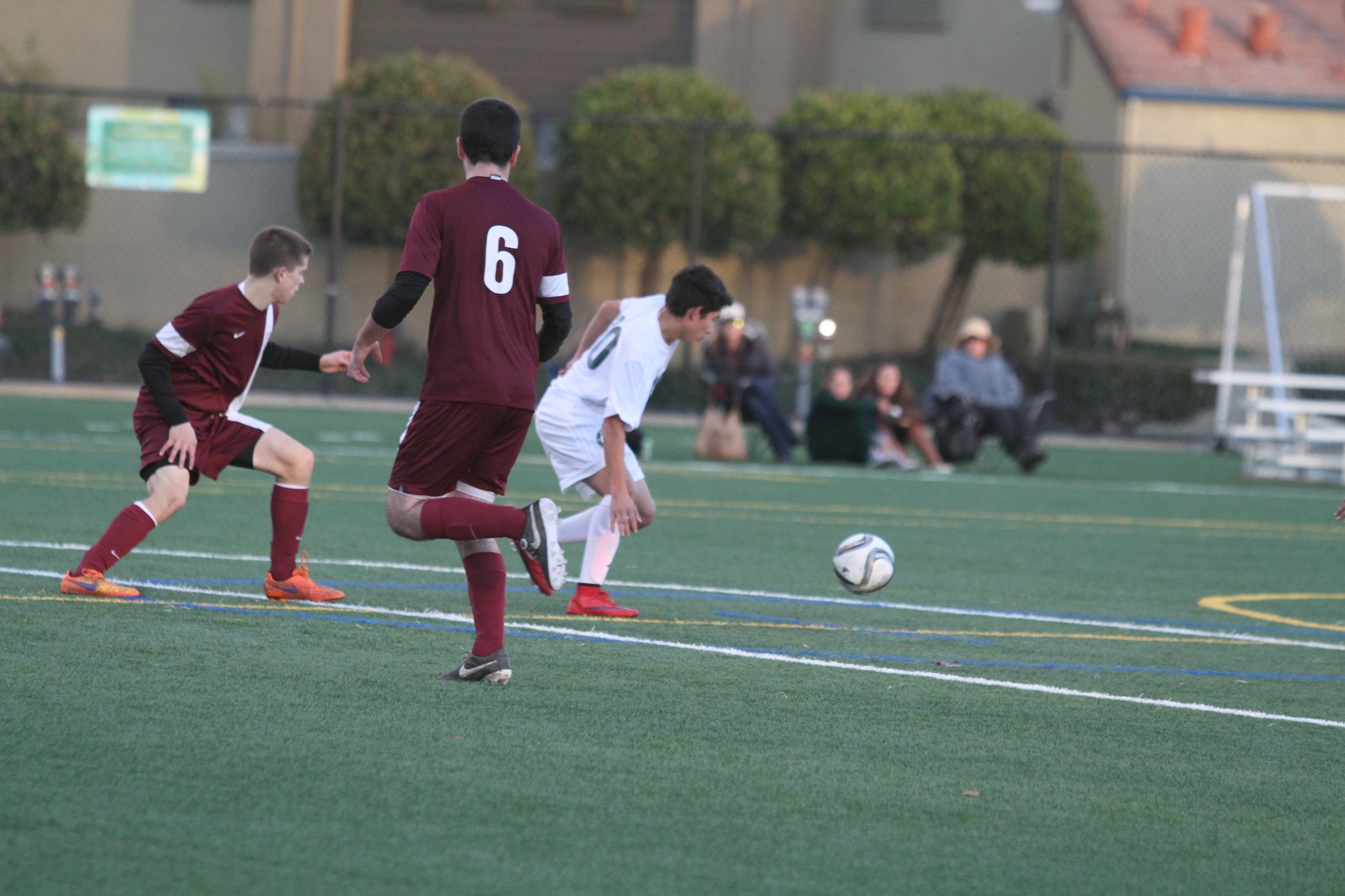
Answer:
[440,646,512,685]
[264,551,345,603]
[566,584,639,617]
[510,537,553,596]
[61,568,140,597]
[518,498,569,590]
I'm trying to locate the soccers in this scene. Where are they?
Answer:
[831,531,895,595]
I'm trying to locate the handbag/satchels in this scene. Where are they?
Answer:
[697,382,747,463]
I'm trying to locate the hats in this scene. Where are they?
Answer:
[954,318,1001,352]
[718,302,746,322]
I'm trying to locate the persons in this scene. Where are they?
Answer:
[694,303,806,466]
[347,98,573,684]
[807,361,919,470]
[855,363,954,477]
[924,316,1050,475]
[59,226,346,603]
[532,264,735,618]
